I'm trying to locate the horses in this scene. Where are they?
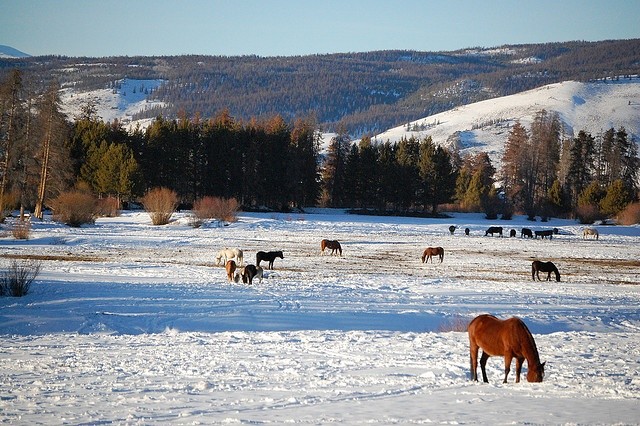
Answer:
[510,229,516,237]
[582,229,599,240]
[226,260,236,283]
[532,260,561,282]
[521,228,533,238]
[534,230,553,240]
[512,230,514,233]
[321,239,342,257]
[486,227,503,238]
[215,247,243,268]
[241,264,257,285]
[449,226,456,235]
[234,266,263,283]
[256,251,284,270]
[422,247,444,264]
[467,314,546,384]
[553,228,558,235]
[465,228,470,235]
